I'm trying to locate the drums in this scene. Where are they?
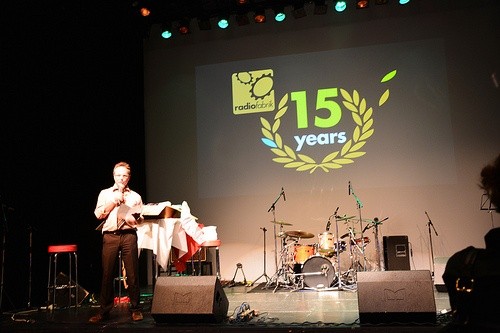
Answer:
[302,256,335,289]
[317,232,335,255]
[293,244,316,263]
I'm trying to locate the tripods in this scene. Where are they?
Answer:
[250,193,325,294]
[331,207,371,289]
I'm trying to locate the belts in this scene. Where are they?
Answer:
[104,230,137,234]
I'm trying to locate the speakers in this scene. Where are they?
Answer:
[150,277,229,324]
[171,246,207,262]
[51,272,89,306]
[383,235,410,271]
[357,270,437,325]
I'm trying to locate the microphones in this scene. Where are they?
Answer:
[117,183,124,206]
[362,224,369,233]
[323,265,327,276]
[282,187,286,200]
[349,181,350,195]
[326,219,330,231]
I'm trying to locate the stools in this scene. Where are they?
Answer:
[43,244,80,310]
[192,239,221,276]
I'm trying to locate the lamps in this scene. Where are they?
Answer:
[235,14,250,27]
[160,20,175,42]
[292,3,307,18]
[272,7,287,25]
[198,16,211,33]
[178,17,193,35]
[313,0,410,15]
[216,14,233,31]
[252,7,268,25]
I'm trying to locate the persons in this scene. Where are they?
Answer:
[89,162,143,322]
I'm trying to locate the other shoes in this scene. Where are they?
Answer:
[88,314,104,324]
[131,311,143,321]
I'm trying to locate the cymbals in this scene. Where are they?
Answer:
[285,231,315,239]
[270,220,294,226]
[340,232,356,239]
[336,215,356,222]
[358,218,372,223]
